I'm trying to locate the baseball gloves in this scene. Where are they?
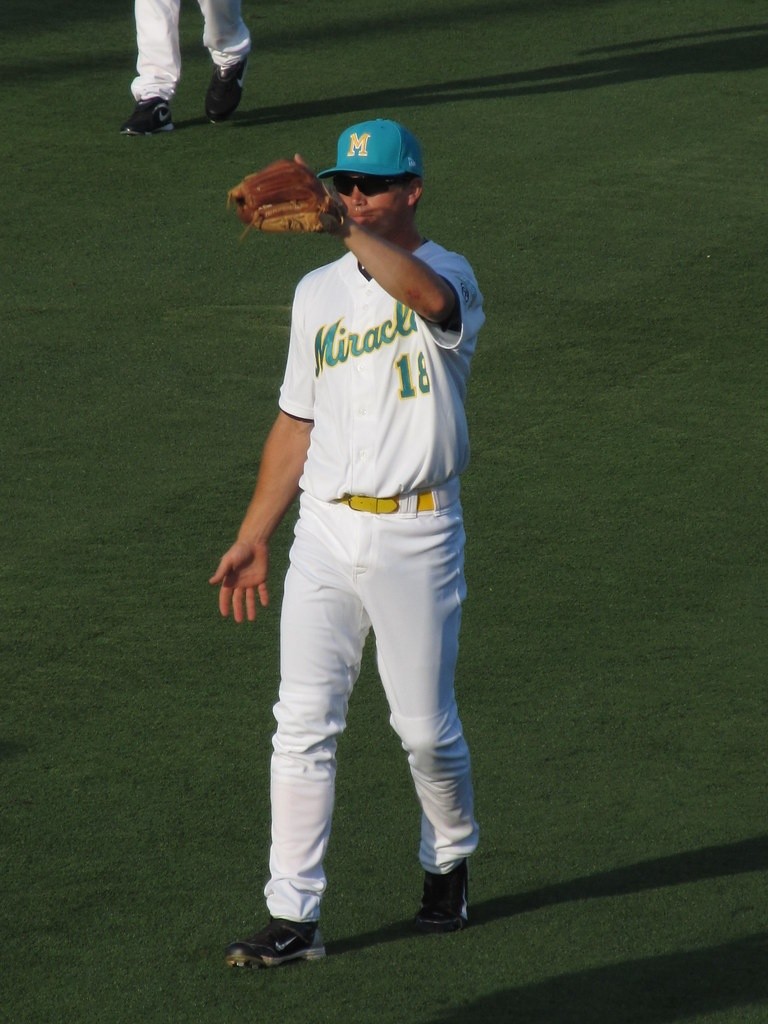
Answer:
[225,162,350,236]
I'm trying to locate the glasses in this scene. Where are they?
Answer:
[329,174,401,196]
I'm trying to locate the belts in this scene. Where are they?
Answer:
[337,491,437,514]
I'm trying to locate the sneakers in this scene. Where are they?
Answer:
[413,859,471,934]
[119,96,175,137]
[205,58,247,120]
[224,917,328,967]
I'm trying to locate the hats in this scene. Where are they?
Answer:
[316,118,425,181]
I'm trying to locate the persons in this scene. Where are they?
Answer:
[206,117,485,967]
[120,0,250,136]
